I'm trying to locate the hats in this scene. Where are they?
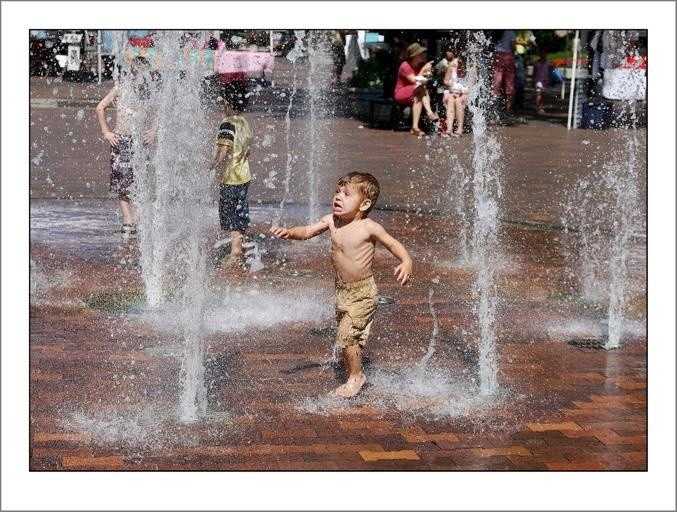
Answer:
[406,42,428,58]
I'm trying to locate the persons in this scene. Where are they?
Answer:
[125,29,299,58]
[199,82,251,268]
[530,46,557,117]
[95,55,160,236]
[268,171,412,399]
[392,29,537,137]
[328,33,346,88]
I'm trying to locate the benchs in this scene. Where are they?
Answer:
[356,66,407,132]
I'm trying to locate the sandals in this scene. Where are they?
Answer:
[410,114,464,139]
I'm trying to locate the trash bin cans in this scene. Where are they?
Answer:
[582,102,612,130]
